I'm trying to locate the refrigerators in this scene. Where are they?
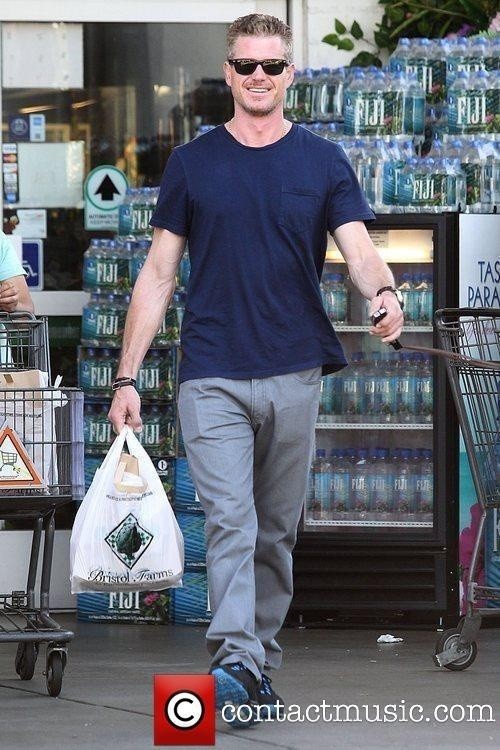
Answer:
[285,214,500,628]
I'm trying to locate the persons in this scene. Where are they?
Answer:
[105,13,406,728]
[1,226,36,328]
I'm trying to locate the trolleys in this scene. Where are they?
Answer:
[428,309,500,672]
[0,309,87,695]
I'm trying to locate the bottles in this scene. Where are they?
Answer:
[304,271,434,524]
[197,124,210,137]
[75,188,191,505]
[283,34,500,216]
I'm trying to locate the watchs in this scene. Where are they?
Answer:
[375,285,405,310]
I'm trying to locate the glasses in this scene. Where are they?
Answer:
[228,58,290,77]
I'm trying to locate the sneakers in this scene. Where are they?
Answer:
[210,661,283,726]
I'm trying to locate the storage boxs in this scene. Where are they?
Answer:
[74,585,172,627]
[173,507,208,568]
[173,457,208,510]
[167,570,213,624]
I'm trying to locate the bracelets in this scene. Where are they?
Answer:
[110,377,137,391]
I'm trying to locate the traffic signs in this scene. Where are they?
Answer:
[82,163,129,231]
